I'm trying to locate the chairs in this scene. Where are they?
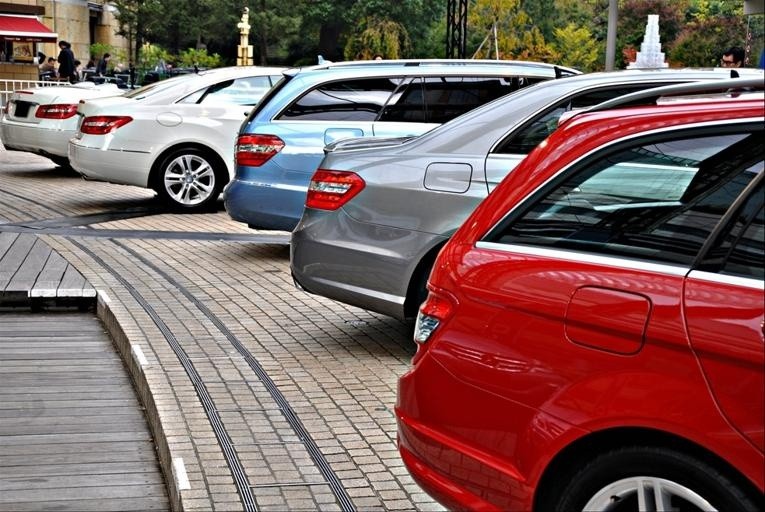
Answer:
[39,61,160,93]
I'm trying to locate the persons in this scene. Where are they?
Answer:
[57,40,76,83]
[113,62,123,73]
[96,53,111,79]
[372,54,383,61]
[718,46,745,68]
[37,51,96,87]
